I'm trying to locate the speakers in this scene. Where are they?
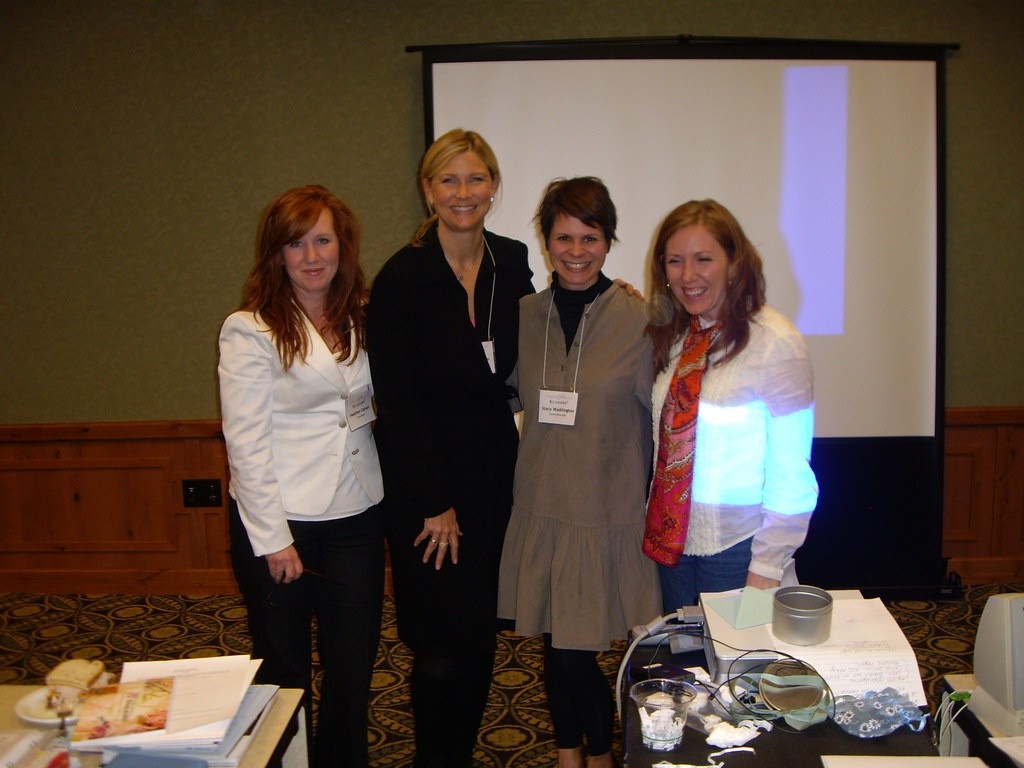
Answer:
[968,595,1024,739]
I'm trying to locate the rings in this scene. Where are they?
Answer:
[430,538,438,543]
[364,128,537,768]
[439,542,449,549]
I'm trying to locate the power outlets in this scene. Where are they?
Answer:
[182,479,222,508]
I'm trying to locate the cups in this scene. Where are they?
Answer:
[629,678,698,753]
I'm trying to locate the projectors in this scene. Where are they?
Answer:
[698,590,865,691]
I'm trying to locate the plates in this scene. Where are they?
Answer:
[15,687,81,726]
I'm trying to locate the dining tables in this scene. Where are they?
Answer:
[0,685,307,768]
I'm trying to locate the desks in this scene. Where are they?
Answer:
[940,673,1024,768]
[622,628,940,768]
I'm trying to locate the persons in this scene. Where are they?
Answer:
[641,198,819,614]
[496,174,666,768]
[219,184,388,768]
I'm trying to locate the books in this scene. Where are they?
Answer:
[70,674,174,744]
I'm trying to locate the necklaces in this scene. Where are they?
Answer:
[440,238,484,281]
[294,297,334,336]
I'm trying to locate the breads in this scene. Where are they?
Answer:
[45,658,105,690]
